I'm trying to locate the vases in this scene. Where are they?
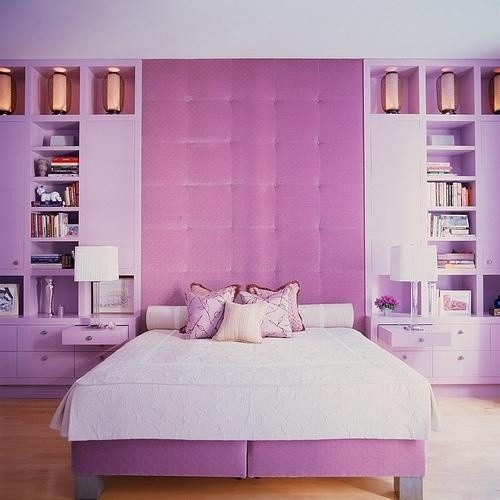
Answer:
[384,309,392,316]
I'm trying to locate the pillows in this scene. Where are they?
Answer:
[145,281,355,343]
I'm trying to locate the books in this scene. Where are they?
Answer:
[427,181,472,206]
[31,201,63,207]
[64,182,79,207]
[31,212,68,238]
[437,253,476,268]
[428,282,439,316]
[427,161,455,174]
[430,213,441,236]
[48,156,79,177]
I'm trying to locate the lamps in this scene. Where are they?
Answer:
[389,246,438,329]
[74,246,119,324]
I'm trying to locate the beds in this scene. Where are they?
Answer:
[66,327,435,500]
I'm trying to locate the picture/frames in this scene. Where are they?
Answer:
[0,284,21,316]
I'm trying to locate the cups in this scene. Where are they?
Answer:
[39,278,54,318]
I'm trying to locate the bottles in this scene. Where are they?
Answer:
[494,295,500,309]
[56,303,64,317]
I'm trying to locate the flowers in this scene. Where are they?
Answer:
[373,294,401,312]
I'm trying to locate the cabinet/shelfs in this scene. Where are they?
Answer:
[362,58,499,386]
[0,58,141,401]
